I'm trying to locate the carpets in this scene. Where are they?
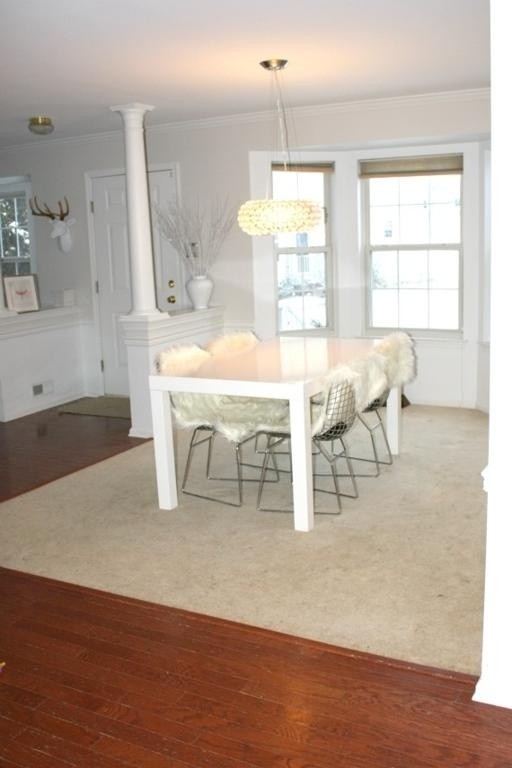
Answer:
[0,399,487,680]
[56,394,132,420]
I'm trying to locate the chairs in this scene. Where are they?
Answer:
[157,329,322,511]
[252,335,418,517]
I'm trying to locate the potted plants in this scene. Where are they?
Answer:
[147,194,239,312]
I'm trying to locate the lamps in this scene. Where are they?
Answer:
[24,116,56,137]
[235,57,320,238]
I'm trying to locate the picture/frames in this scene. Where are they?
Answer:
[1,271,42,314]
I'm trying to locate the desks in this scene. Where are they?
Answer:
[145,333,409,533]
